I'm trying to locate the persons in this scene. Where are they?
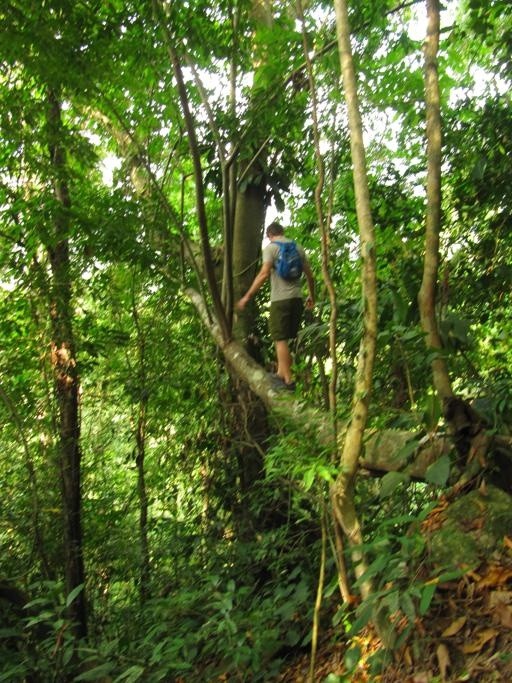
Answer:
[237,223,314,391]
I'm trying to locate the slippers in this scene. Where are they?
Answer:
[271,239,301,281]
